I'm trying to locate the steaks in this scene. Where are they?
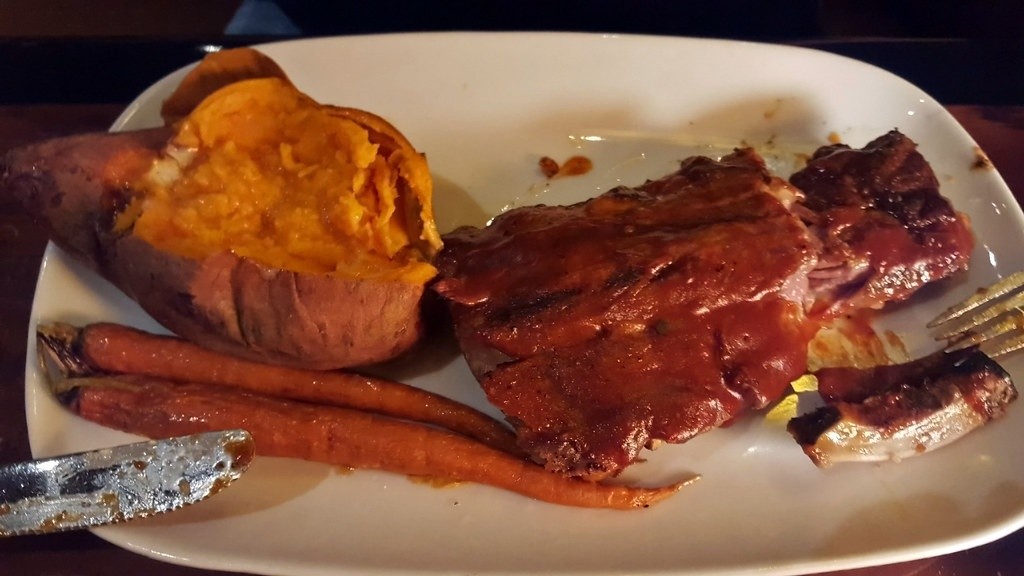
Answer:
[432,129,1018,480]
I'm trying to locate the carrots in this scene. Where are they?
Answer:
[36,353,702,510]
[36,322,531,459]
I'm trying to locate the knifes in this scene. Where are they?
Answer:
[0,421,250,544]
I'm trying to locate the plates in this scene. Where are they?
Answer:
[17,24,1023,574]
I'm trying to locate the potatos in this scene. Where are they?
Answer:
[0,47,446,370]
